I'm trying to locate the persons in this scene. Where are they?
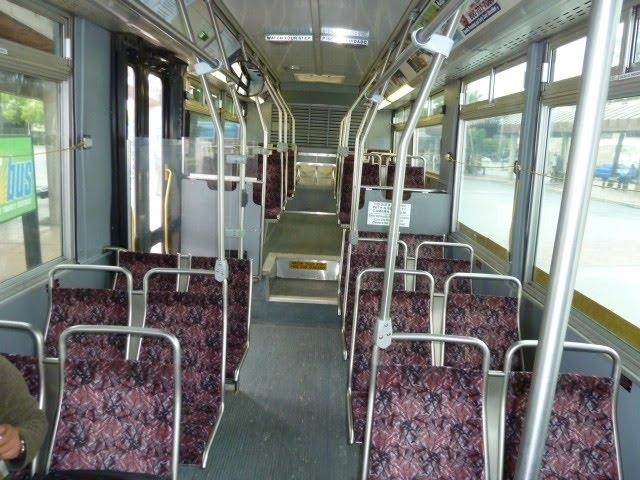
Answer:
[0,352,48,480]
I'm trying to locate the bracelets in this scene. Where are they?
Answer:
[17,433,26,456]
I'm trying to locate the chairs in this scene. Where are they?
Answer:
[347,267,435,447]
[197,145,298,225]
[361,332,491,480]
[112,248,181,295]
[334,146,428,230]
[497,339,624,480]
[1,319,45,480]
[44,263,134,364]
[46,324,181,479]
[335,229,475,362]
[186,254,253,382]
[441,272,526,372]
[135,267,228,469]
